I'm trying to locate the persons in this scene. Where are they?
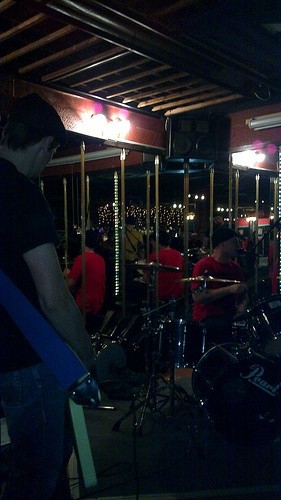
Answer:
[62,230,105,316]
[113,215,143,289]
[0,92,96,500]
[206,214,281,294]
[191,227,249,349]
[137,228,183,300]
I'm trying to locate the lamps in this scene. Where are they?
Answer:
[245,112,281,131]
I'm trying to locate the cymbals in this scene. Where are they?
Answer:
[127,262,180,272]
[179,276,242,283]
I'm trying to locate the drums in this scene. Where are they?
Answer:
[191,342,281,441]
[156,318,207,368]
[245,295,281,356]
[86,333,127,381]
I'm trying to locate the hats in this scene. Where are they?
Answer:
[7,92,68,146]
[86,232,97,248]
[126,217,136,225]
[212,228,239,249]
[160,233,172,245]
[191,233,198,237]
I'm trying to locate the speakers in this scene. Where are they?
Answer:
[168,114,230,161]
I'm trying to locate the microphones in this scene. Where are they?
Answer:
[238,248,261,257]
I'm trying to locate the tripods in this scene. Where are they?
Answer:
[112,268,207,437]
